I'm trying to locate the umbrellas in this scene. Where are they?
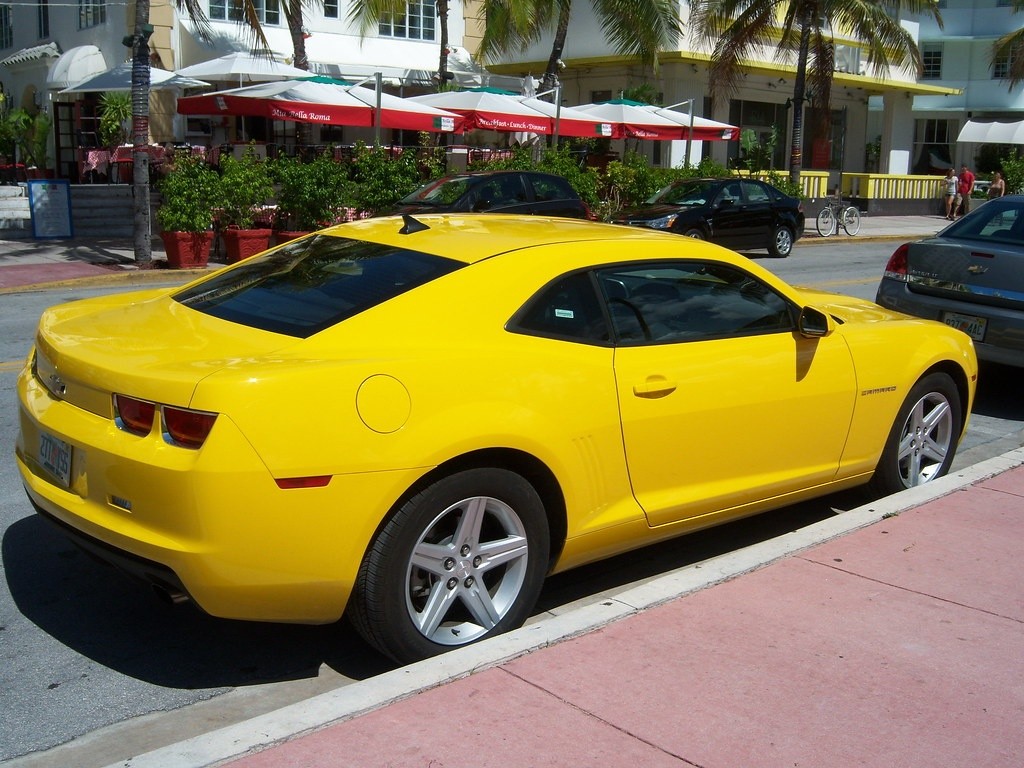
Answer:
[56,58,175,142]
[177,51,314,144]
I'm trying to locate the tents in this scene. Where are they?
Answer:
[177,75,739,170]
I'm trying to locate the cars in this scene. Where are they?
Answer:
[11,213,972,665]
[875,194,1024,370]
[606,176,805,257]
[367,169,594,220]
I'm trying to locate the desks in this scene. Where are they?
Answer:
[113,145,164,183]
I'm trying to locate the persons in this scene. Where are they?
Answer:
[944,168,958,219]
[948,163,974,221]
[987,171,1004,199]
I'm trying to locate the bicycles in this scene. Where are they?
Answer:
[815,196,861,238]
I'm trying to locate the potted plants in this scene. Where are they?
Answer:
[217,139,274,262]
[158,149,224,268]
[265,142,345,246]
[963,191,989,215]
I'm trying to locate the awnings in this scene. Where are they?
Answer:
[490,76,540,93]
[955,116,1024,145]
[180,18,490,88]
[46,45,107,89]
